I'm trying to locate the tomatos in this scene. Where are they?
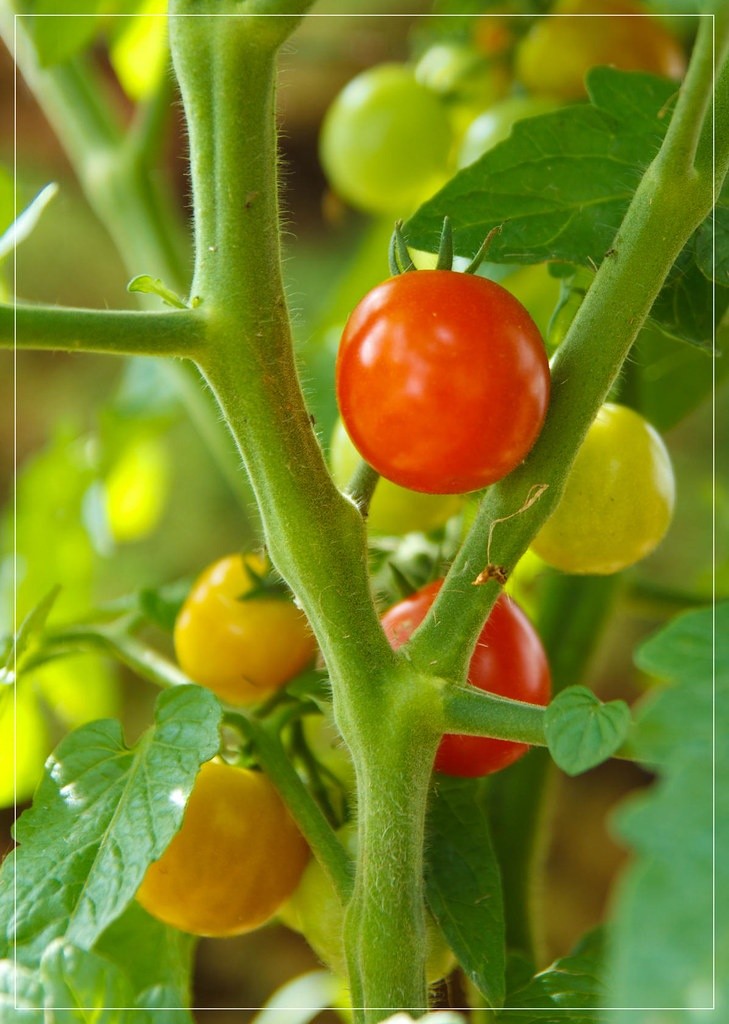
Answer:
[1,2,725,994]
